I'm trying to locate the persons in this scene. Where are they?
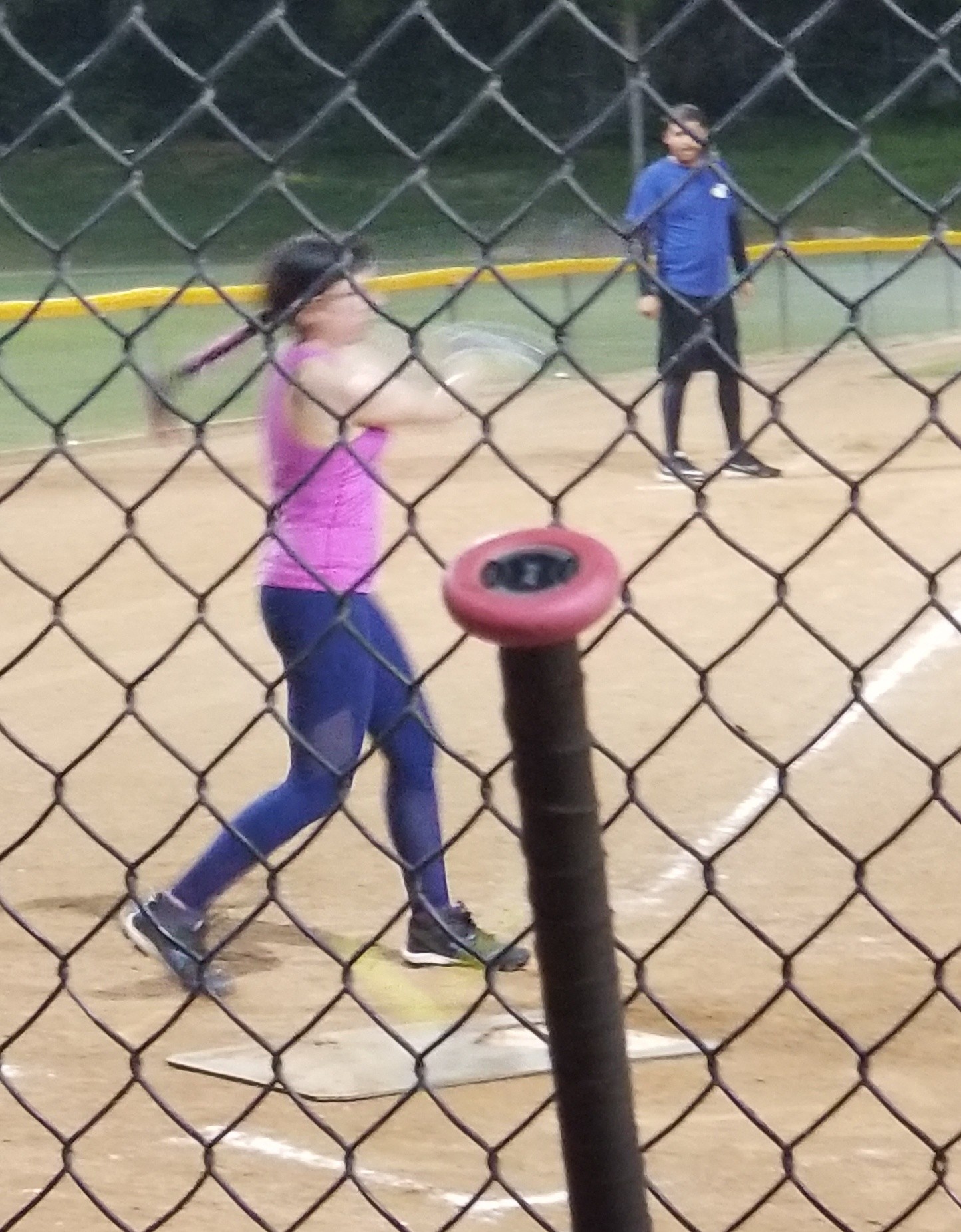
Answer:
[120,234,532,995]
[622,103,785,480]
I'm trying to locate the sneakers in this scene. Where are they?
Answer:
[122,890,234,994]
[658,451,708,480]
[721,449,781,478]
[402,900,531,970]
